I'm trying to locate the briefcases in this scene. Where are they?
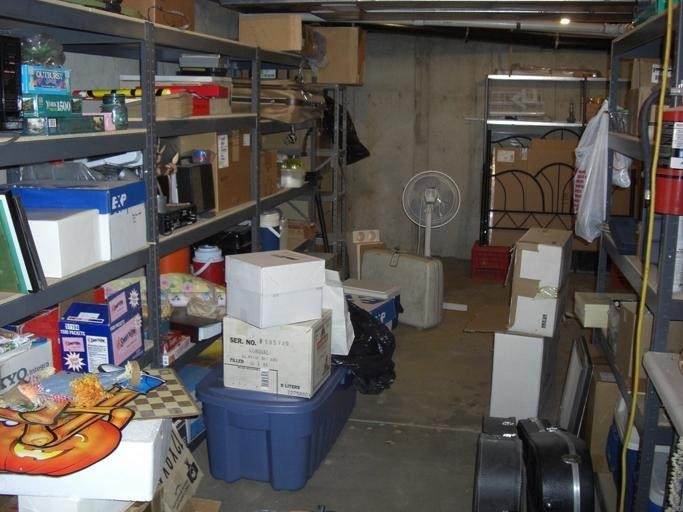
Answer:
[361,248,443,330]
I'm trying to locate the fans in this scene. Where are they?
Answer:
[402,170,470,311]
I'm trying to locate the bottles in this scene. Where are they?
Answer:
[101,94,128,130]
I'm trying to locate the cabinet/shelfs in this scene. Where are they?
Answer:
[573,0,683,512]
[0,0,349,446]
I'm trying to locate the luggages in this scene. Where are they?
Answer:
[231,80,328,124]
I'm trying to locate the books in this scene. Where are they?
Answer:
[0,186,50,297]
[167,310,224,343]
[176,52,231,76]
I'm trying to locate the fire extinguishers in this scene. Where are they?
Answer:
[640,81,683,216]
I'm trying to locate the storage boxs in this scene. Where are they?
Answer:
[0,180,148,396]
[237,13,302,52]
[463,57,683,512]
[343,277,401,330]
[225,250,325,329]
[196,361,358,492]
[177,126,279,211]
[121,0,196,32]
[304,25,366,86]
